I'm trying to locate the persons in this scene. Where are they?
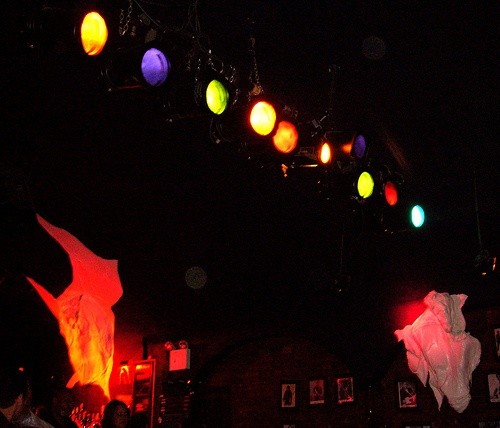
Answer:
[38,387,77,428]
[1,369,30,428]
[100,398,130,427]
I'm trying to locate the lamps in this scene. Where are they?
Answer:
[28,2,432,233]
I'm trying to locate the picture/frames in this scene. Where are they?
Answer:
[277,328,500,411]
